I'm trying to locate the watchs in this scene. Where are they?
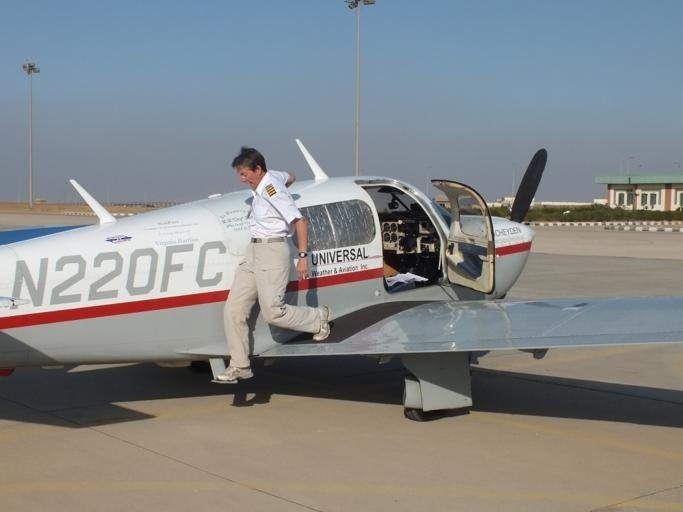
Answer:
[297,252,306,258]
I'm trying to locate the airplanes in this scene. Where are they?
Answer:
[0,147,681,422]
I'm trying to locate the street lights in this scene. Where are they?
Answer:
[342,0,375,176]
[22,62,41,210]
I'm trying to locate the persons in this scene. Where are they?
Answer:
[213,145,331,383]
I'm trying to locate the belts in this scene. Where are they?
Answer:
[251,237,284,243]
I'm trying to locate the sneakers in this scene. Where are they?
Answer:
[216,366,254,381]
[313,305,331,341]
